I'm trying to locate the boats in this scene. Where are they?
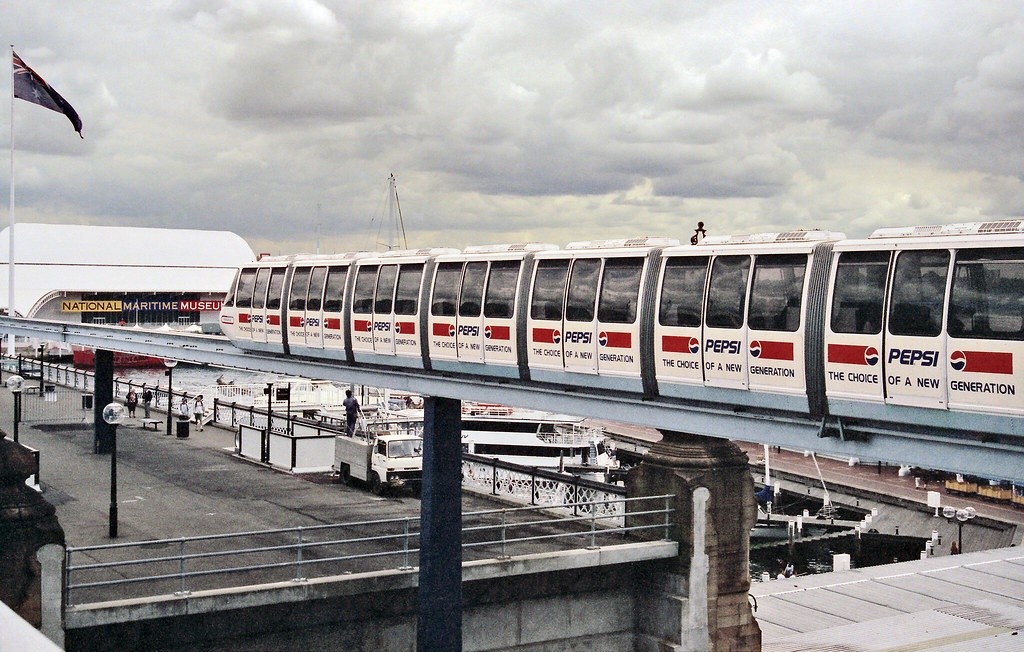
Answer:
[393,402,621,482]
[72,348,165,369]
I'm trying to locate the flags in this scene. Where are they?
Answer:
[13,51,83,138]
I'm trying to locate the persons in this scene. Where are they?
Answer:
[195,396,203,432]
[142,389,152,418]
[343,390,365,438]
[756,486,770,505]
[180,398,189,415]
[126,388,138,418]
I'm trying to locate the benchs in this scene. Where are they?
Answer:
[24,386,42,394]
[137,419,163,432]
[4,380,8,387]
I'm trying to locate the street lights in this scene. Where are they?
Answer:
[163,357,178,435]
[103,402,125,538]
[941,505,977,555]
[7,374,27,443]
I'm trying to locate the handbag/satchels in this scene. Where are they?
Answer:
[124,400,130,406]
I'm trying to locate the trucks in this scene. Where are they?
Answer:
[332,432,424,495]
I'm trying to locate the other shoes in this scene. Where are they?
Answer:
[200,428,203,432]
[133,416,136,418]
[195,428,198,431]
[144,416,150,418]
[129,415,131,418]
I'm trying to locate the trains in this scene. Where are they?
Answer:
[218,215,1024,444]
